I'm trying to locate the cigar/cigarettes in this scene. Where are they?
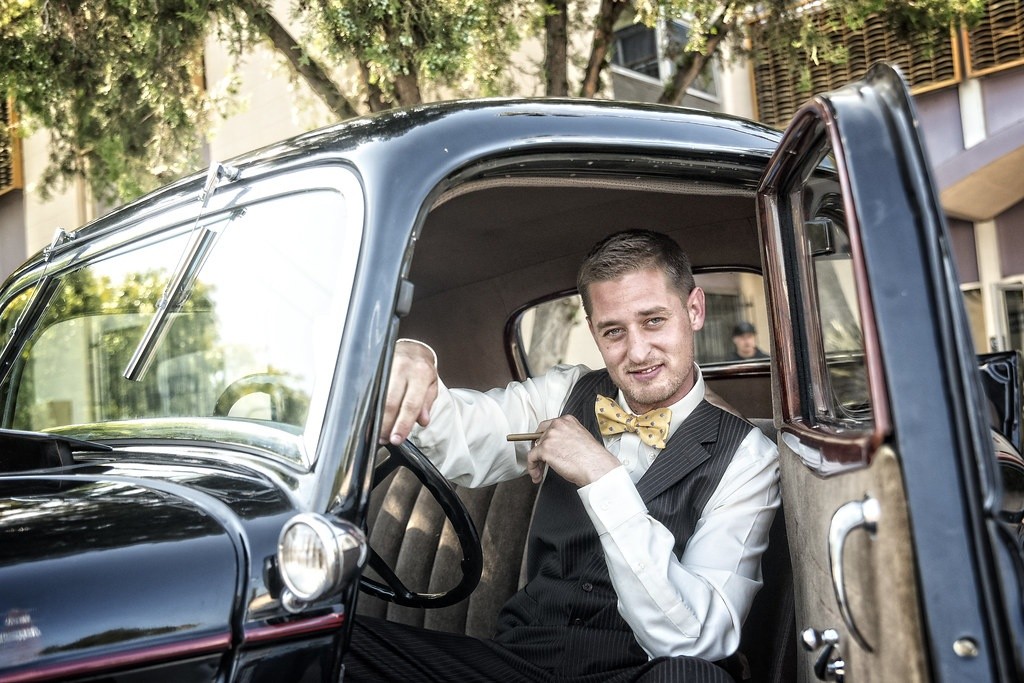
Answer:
[507,433,543,441]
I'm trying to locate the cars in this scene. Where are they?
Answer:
[0,57,1024,683]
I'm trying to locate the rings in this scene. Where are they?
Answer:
[534,438,539,447]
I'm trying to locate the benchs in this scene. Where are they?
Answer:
[354,417,776,642]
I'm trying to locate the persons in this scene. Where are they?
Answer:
[725,323,770,361]
[339,227,778,681]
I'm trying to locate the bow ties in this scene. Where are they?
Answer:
[594,394,672,449]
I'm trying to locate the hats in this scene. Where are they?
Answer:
[732,323,755,336]
